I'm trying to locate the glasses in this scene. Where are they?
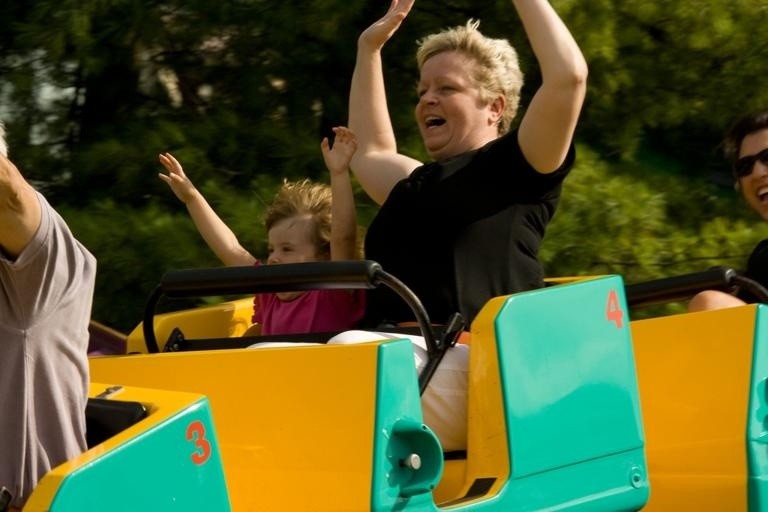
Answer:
[735,149,768,176]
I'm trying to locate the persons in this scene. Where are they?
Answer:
[687,110,768,313]
[0,120,97,512]
[159,125,366,336]
[245,1,590,453]
